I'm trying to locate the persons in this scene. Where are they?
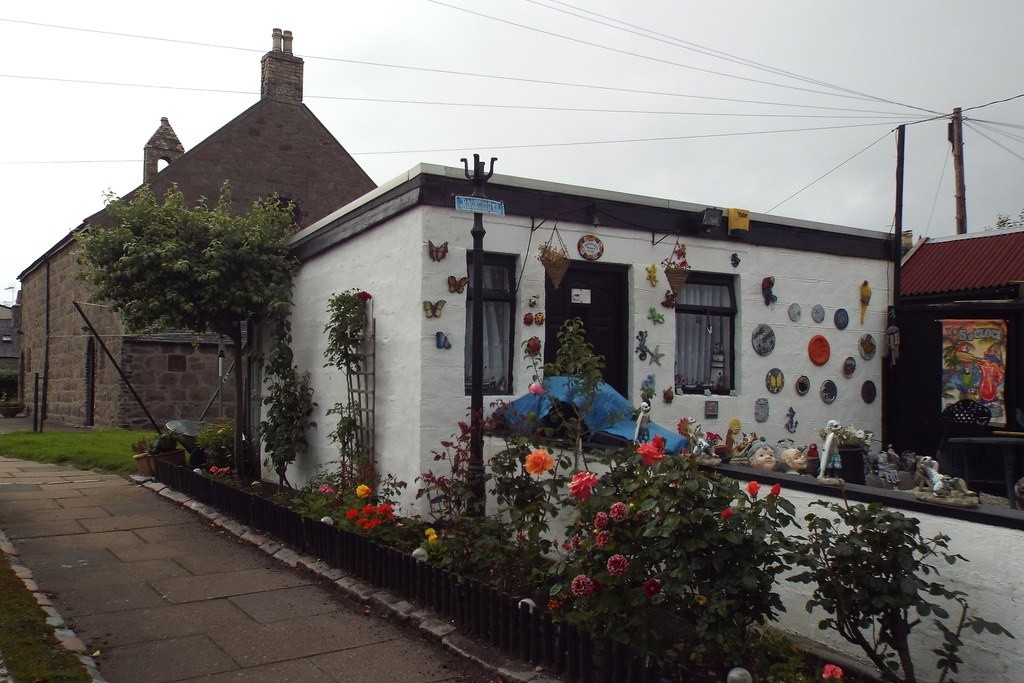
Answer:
[747,441,776,470]
[780,449,807,475]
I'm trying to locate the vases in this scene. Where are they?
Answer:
[663,267,689,296]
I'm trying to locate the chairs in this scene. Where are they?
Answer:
[936,399,992,504]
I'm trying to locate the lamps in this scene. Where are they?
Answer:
[696,207,723,227]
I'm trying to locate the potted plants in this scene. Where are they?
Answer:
[537,223,571,289]
[149,430,185,474]
[131,438,148,475]
[0,392,25,418]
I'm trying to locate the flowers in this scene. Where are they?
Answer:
[660,242,690,268]
